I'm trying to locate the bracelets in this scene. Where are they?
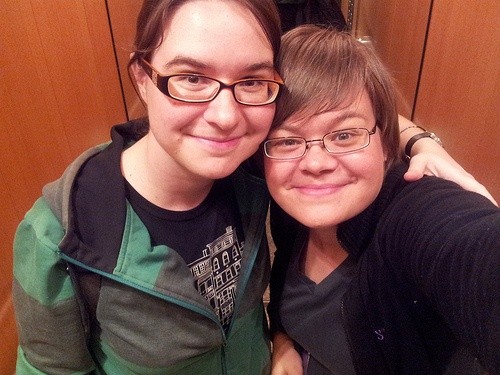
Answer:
[398,126,429,132]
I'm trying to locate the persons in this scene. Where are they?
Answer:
[13,0,500,375]
[262,26,498,375]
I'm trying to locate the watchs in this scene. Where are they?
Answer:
[404,132,448,159]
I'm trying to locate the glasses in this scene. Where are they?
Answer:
[261,123,377,160]
[138,56,284,107]
